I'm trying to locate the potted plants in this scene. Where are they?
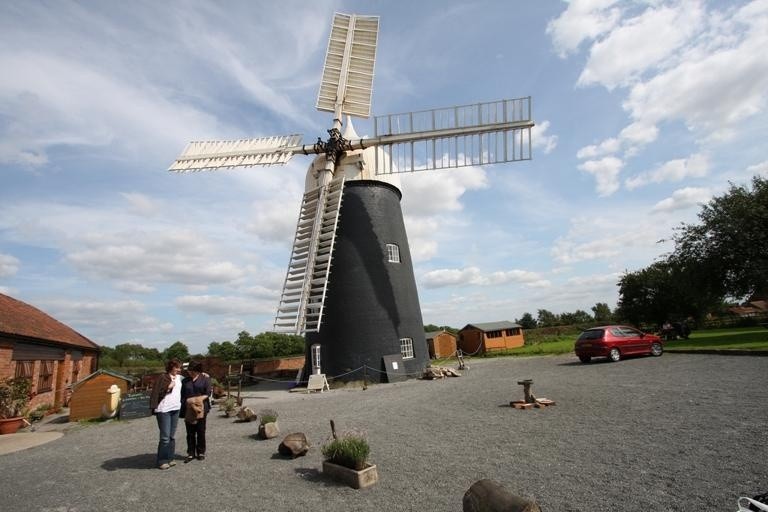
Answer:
[0,375,35,434]
[320,431,381,489]
[224,398,239,417]
[258,410,282,438]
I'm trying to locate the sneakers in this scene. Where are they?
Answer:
[198,453,205,461]
[159,460,176,470]
[184,455,196,463]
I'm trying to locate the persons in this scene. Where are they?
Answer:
[150,357,210,469]
[661,319,690,339]
[179,361,213,462]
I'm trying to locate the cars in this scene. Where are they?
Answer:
[574,325,663,362]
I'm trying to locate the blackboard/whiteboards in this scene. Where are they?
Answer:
[119,391,152,420]
